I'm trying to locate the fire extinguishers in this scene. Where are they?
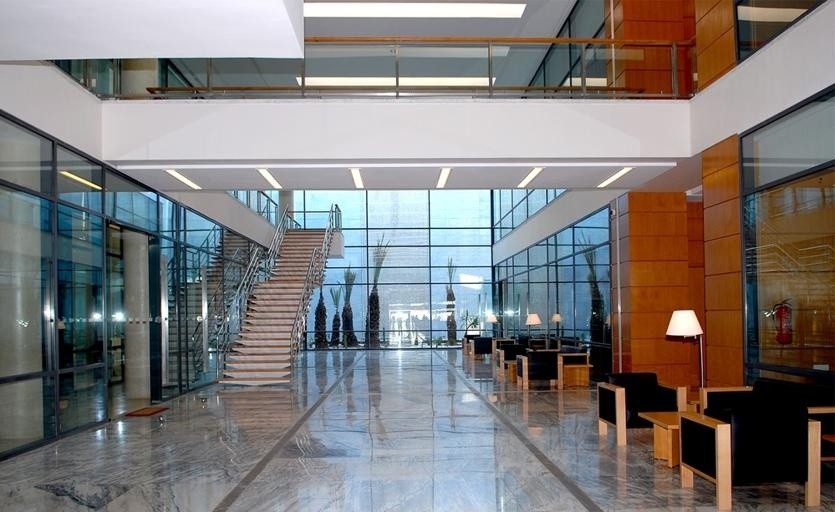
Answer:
[772,297,793,344]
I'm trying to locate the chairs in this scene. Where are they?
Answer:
[596,370,823,511]
[462,334,594,391]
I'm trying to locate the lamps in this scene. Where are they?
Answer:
[667,309,705,391]
[486,314,502,338]
[525,313,542,337]
